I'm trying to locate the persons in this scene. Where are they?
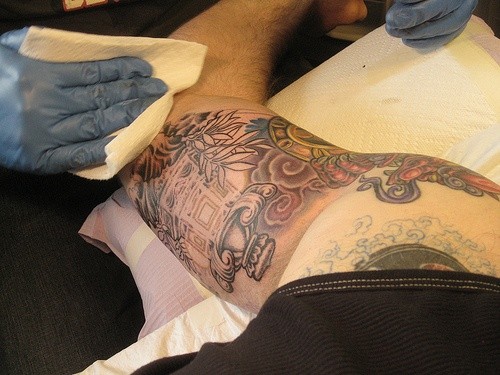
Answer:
[111,0,499,375]
[0,0,479,175]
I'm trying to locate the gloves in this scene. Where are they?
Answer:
[384,0,480,52]
[0,27,168,177]
